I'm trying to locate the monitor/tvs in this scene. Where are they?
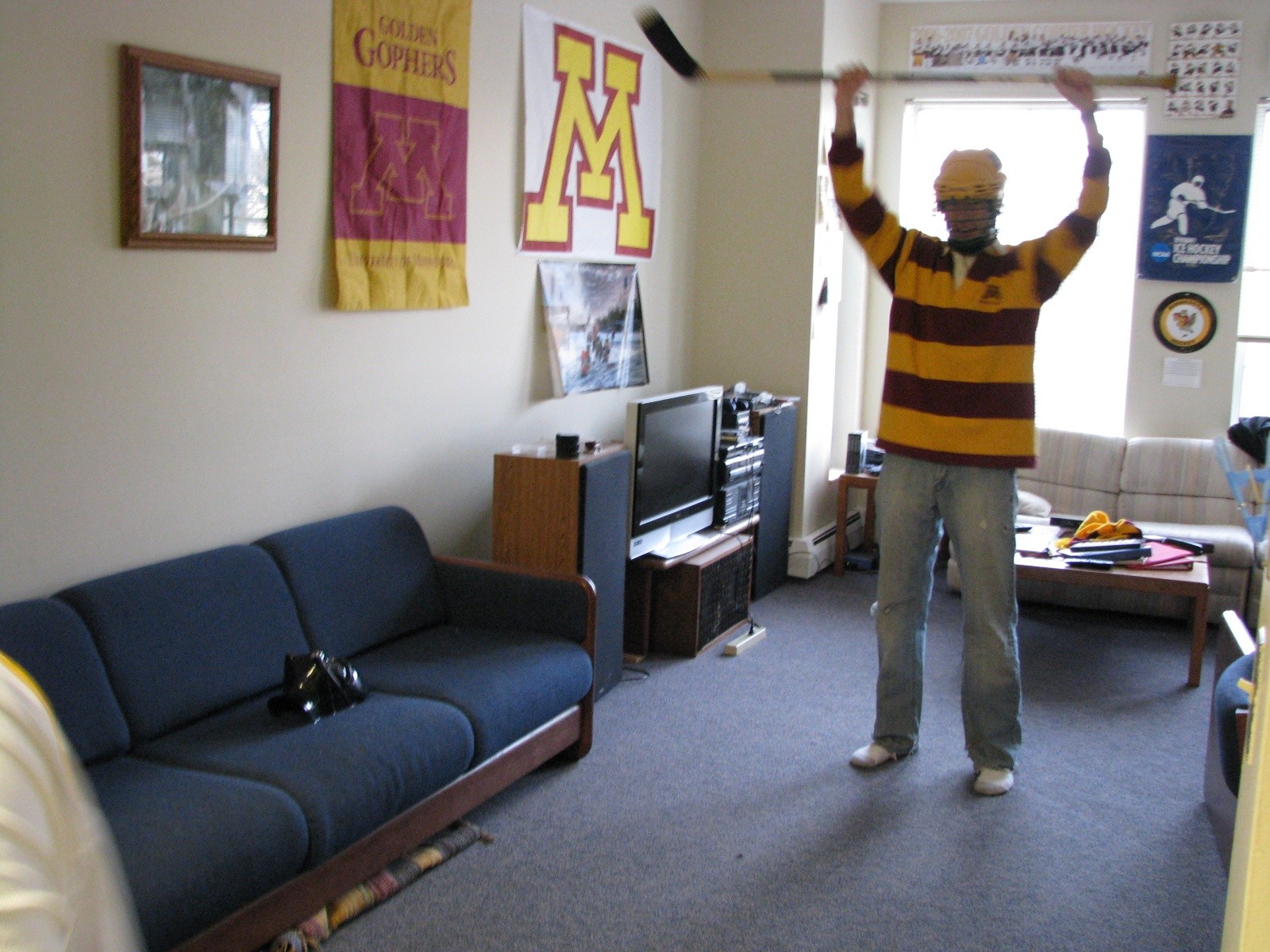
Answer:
[629,383,724,557]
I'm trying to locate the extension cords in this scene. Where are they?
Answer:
[722,626,766,655]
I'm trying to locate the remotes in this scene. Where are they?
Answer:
[1064,538,1152,569]
[1014,525,1032,531]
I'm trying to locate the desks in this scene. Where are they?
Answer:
[1014,539,1211,690]
[627,513,761,661]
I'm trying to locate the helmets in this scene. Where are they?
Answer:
[934,148,1007,253]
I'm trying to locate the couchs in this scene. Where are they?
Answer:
[946,428,1270,630]
[0,504,599,952]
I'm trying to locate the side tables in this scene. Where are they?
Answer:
[834,472,880,577]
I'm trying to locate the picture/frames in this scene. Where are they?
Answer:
[122,43,281,251]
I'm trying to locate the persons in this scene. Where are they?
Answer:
[826,59,1113,797]
[580,324,615,376]
[1150,173,1207,237]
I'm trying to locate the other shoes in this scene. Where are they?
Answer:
[849,741,899,769]
[974,766,1015,794]
